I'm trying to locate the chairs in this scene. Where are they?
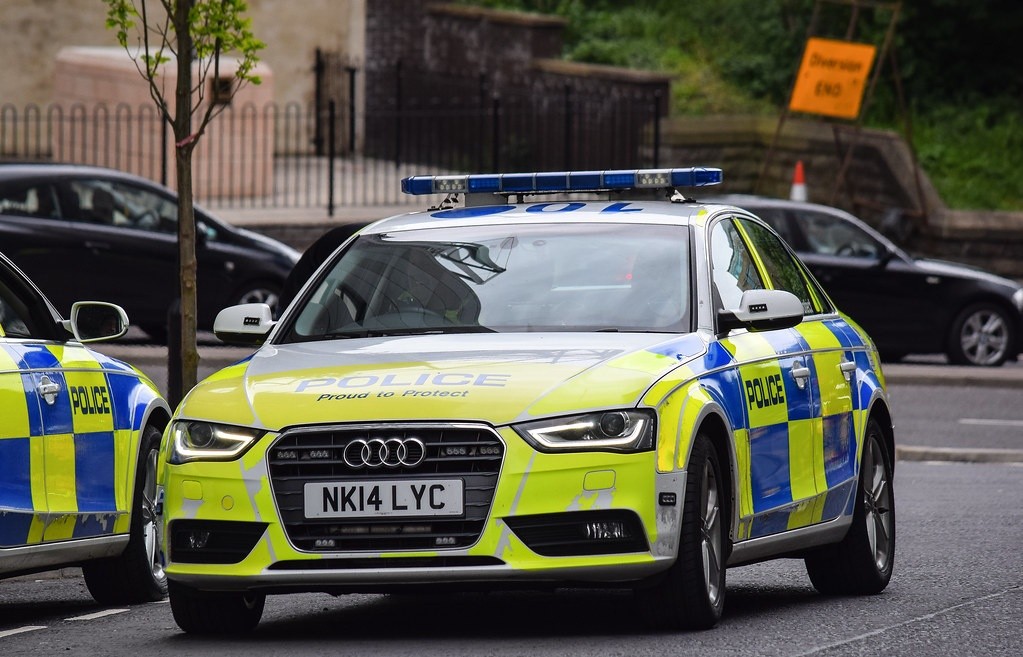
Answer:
[92,187,115,224]
[787,211,818,252]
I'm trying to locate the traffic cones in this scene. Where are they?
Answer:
[791,160,808,204]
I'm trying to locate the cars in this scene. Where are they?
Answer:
[1,251,177,604]
[153,168,899,637]
[0,162,302,347]
[700,195,1023,370]
